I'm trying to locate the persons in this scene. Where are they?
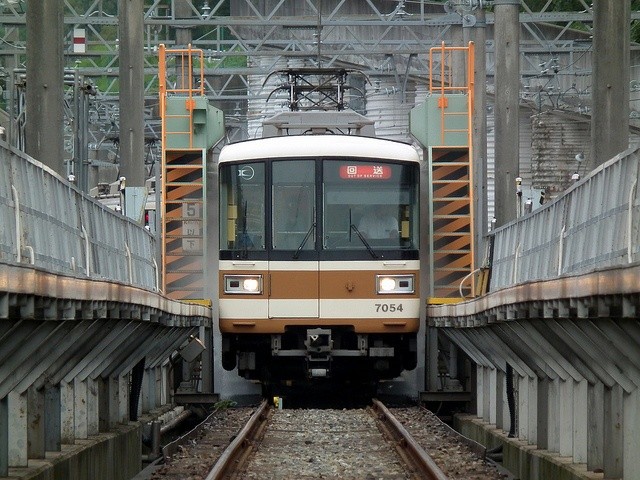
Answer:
[355,205,400,240]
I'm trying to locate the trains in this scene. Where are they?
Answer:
[217,133,421,400]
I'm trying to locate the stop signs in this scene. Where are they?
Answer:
[74,28,85,52]
